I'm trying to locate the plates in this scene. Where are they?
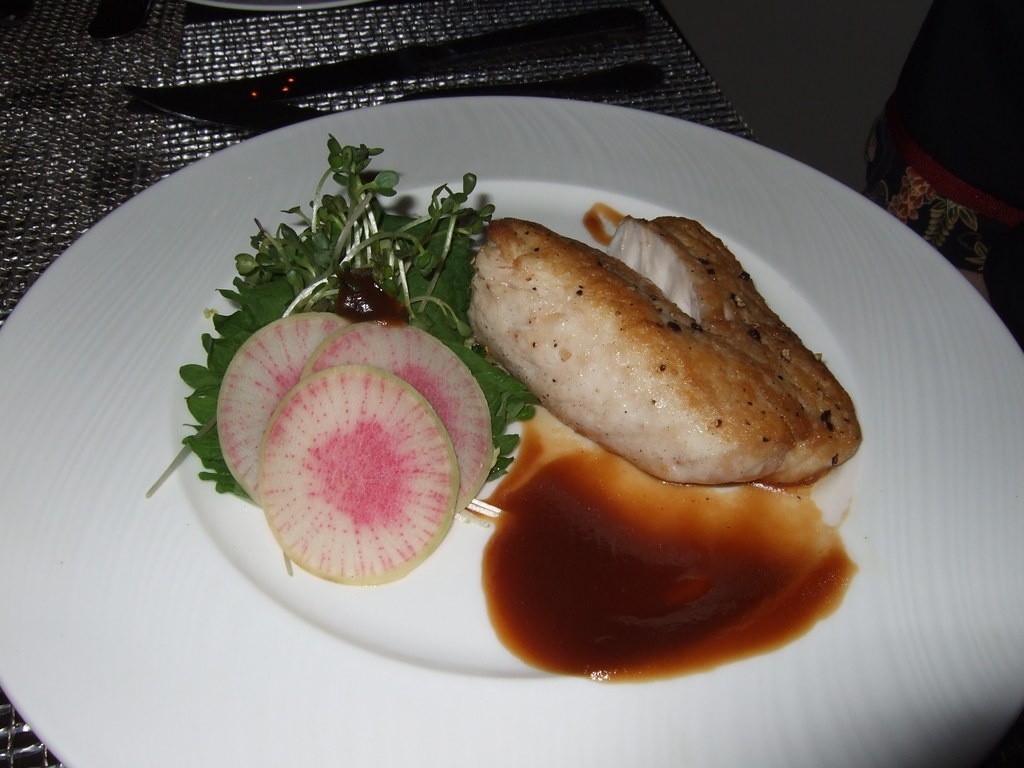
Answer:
[1,94,1024,768]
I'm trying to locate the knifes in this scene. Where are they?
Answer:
[115,2,653,116]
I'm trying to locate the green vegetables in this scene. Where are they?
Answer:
[144,129,543,519]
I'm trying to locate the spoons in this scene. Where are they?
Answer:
[133,58,674,130]
